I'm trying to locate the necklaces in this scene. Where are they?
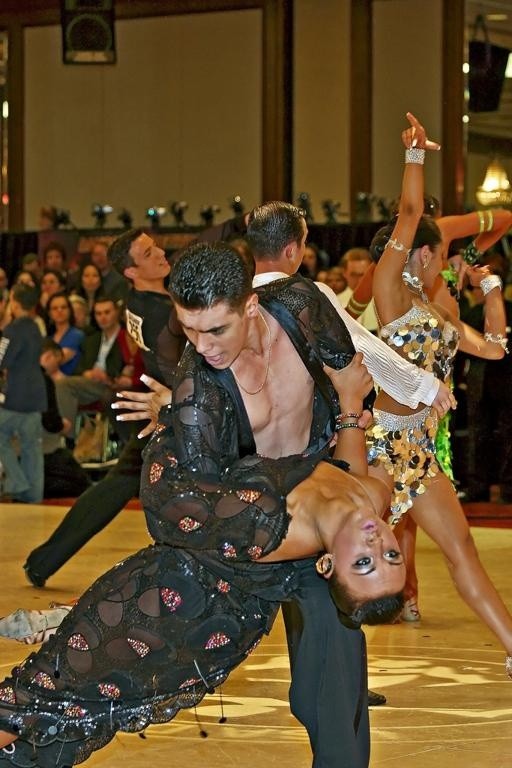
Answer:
[227,306,272,395]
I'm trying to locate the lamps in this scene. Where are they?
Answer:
[321,200,340,225]
[353,194,370,221]
[90,203,112,229]
[169,201,189,225]
[296,191,313,222]
[474,152,510,208]
[201,207,220,225]
[376,196,390,222]
[116,210,132,228]
[144,206,166,230]
[231,196,245,216]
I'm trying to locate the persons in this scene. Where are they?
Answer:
[371,112,512,680]
[1,224,147,506]
[0,238,379,768]
[22,227,188,589]
[0,352,409,768]
[445,245,512,504]
[232,239,371,307]
[341,192,512,322]
[232,199,386,705]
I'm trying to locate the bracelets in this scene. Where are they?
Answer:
[403,148,426,165]
[334,422,366,432]
[333,412,361,423]
[479,274,504,296]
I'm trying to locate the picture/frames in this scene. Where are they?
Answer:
[59,8,118,67]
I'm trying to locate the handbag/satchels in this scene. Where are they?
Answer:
[73,413,106,461]
[14,449,93,501]
